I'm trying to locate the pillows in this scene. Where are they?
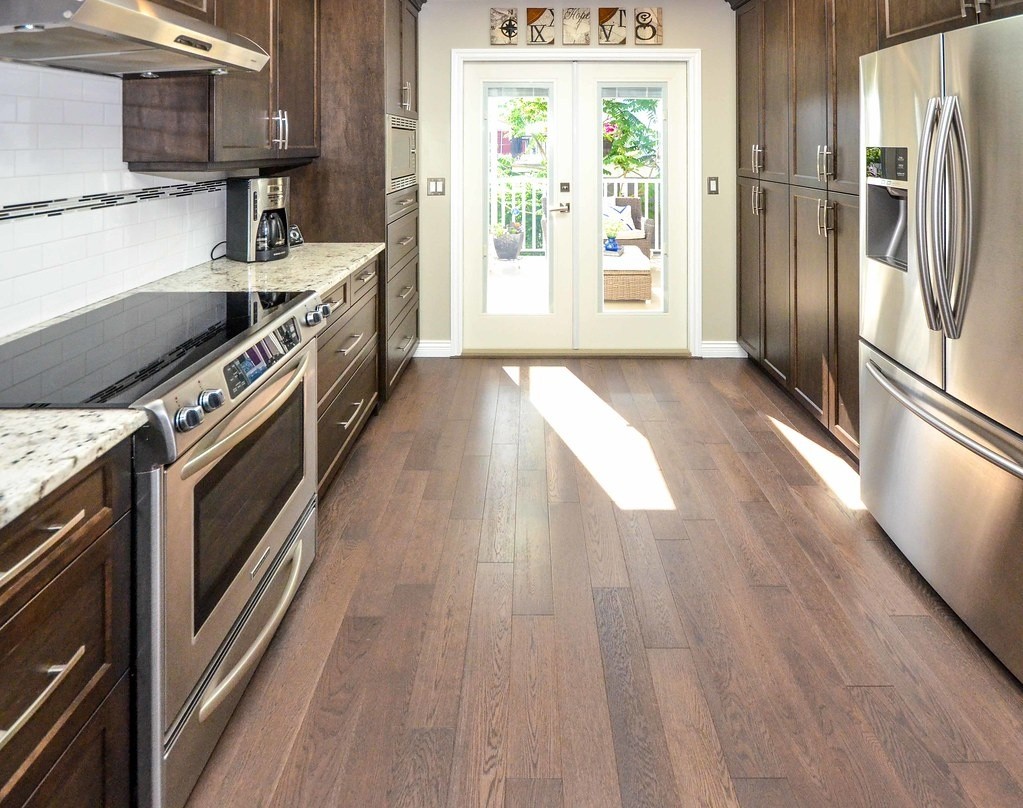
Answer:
[603,206,637,231]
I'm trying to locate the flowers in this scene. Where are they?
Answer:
[603,123,624,143]
[491,209,525,239]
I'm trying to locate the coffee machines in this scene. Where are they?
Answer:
[226,176,290,263]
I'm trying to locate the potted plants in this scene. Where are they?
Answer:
[604,220,623,251]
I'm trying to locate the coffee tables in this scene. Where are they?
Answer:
[603,245,652,300]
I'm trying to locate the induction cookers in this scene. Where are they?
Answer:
[0,291,319,471]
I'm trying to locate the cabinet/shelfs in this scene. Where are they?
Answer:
[289,186,420,400]
[123,0,321,171]
[317,263,381,503]
[319,0,427,117]
[728,1,1023,684]
[0,436,137,808]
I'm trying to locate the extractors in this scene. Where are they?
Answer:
[0,0,273,81]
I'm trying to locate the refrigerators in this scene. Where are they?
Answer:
[859,14,1023,684]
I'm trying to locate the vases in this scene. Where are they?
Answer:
[604,139,614,156]
[493,232,524,260]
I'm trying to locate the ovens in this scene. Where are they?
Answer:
[129,291,316,808]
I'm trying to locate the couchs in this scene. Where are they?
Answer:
[603,197,655,259]
